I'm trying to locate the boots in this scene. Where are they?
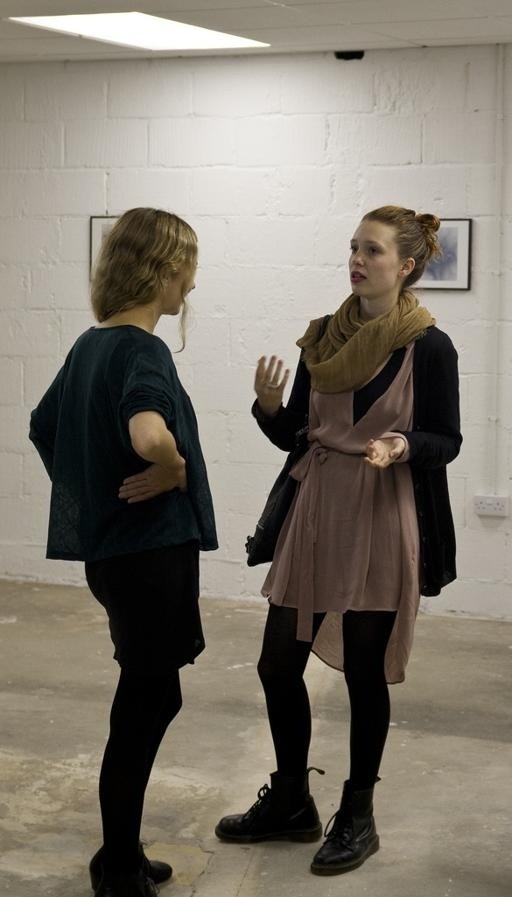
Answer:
[215,767,325,842]
[310,776,380,876]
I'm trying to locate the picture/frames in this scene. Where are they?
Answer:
[90,216,125,282]
[407,219,471,290]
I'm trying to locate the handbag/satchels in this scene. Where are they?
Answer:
[246,424,307,566]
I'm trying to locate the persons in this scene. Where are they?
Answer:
[216,204,464,877]
[29,206,222,897]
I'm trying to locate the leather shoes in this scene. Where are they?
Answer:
[89,841,173,895]
